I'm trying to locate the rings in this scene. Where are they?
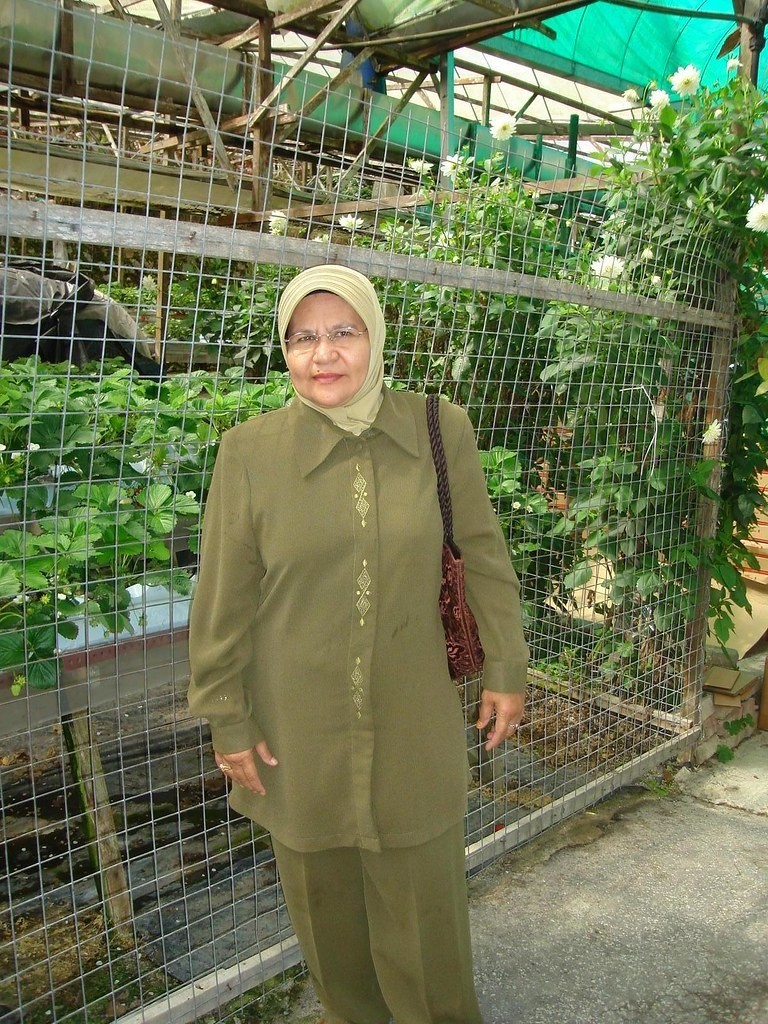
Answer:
[509,723,521,730]
[220,763,231,772]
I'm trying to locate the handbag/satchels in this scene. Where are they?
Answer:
[425,394,487,681]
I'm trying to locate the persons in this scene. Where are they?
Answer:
[186,264,529,1024]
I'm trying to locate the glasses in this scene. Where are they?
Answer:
[284,327,367,352]
